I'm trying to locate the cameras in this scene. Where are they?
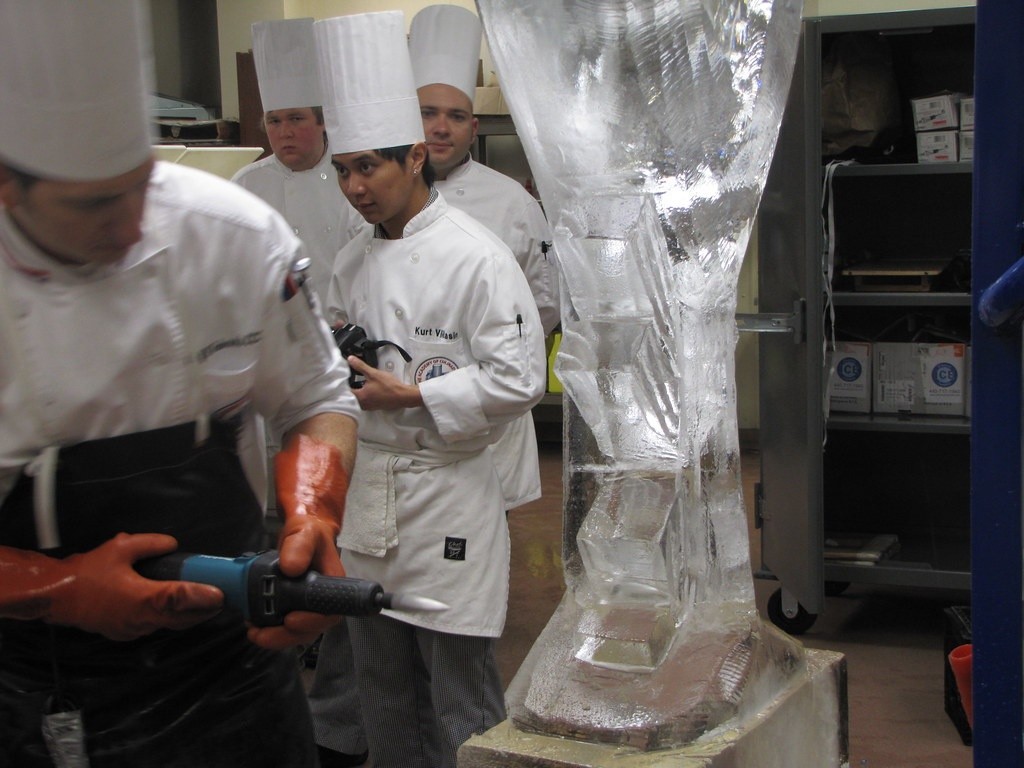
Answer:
[329,323,379,388]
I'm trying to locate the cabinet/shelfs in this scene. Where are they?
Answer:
[752,5,977,635]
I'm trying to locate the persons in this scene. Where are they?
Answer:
[1,0,361,768]
[233,0,560,768]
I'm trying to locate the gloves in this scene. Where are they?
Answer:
[0,533,224,640]
[246,435,346,650]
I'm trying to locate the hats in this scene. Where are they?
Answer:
[312,10,424,154]
[250,17,323,112]
[409,5,483,103]
[0,0,151,181]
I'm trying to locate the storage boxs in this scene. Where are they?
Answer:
[959,132,974,162]
[826,341,872,414]
[872,342,914,415]
[959,97,974,131]
[915,129,959,164]
[910,92,969,132]
[913,343,966,416]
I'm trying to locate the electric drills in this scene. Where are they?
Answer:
[133,549,452,642]
